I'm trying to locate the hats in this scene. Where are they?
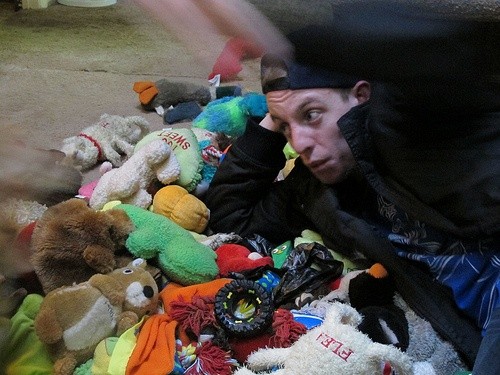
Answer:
[260,25,371,92]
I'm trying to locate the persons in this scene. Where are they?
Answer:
[204,24,500,375]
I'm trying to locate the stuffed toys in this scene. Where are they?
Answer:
[0,31,464,375]
